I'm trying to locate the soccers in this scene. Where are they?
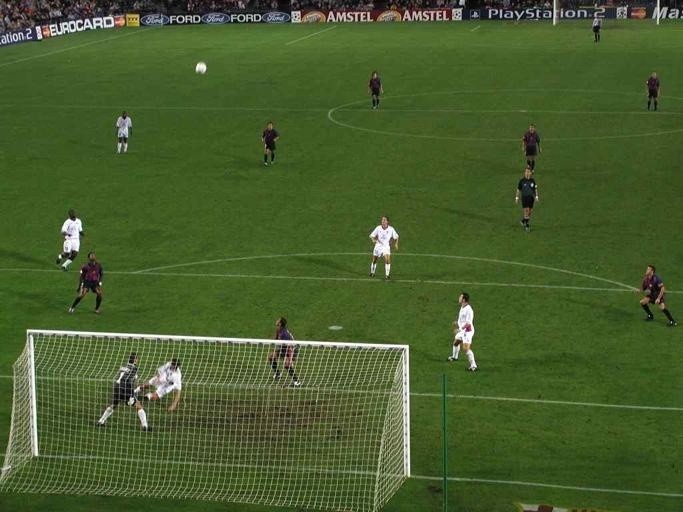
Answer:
[195,62,206,74]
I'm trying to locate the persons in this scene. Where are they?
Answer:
[268,315,302,389]
[523,124,542,175]
[369,214,400,281]
[369,69,383,111]
[1,1,682,43]
[114,111,133,154]
[97,362,152,433]
[55,209,88,271]
[646,73,662,111]
[514,167,539,233]
[69,252,105,315]
[447,291,479,372]
[635,265,677,327]
[261,122,280,167]
[133,358,183,415]
